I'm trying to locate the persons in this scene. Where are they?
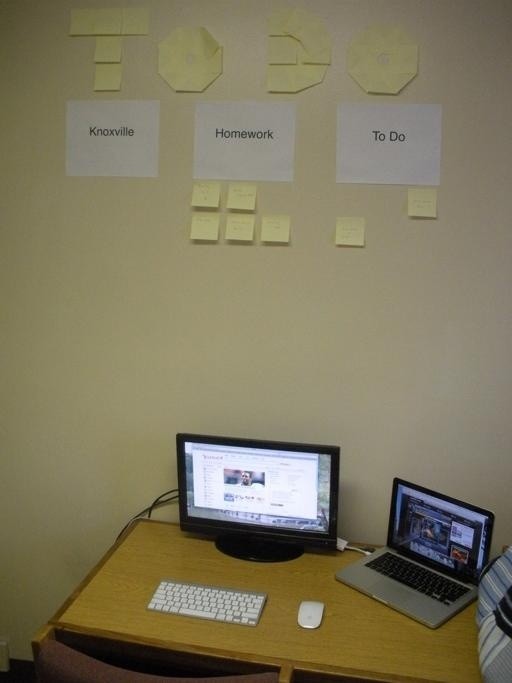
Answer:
[235,470,260,486]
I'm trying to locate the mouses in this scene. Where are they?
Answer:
[297,600,325,629]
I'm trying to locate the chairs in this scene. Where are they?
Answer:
[31,620,294,683]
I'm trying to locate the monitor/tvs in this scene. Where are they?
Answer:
[174,432,340,563]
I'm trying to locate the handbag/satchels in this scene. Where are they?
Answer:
[474,543,512,683]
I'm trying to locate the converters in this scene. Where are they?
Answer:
[337,536,348,552]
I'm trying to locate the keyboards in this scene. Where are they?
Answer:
[147,579,267,628]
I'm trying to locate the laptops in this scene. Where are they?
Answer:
[335,477,494,630]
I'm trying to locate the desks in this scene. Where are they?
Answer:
[46,516,479,683]
